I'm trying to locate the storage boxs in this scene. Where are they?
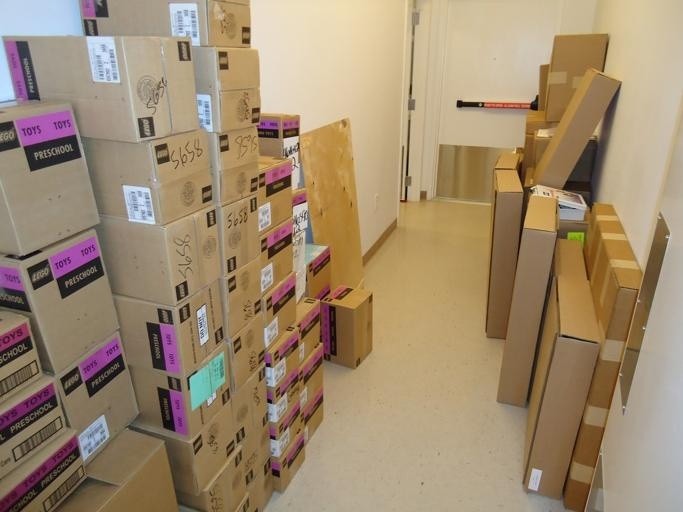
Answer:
[319,285,372,370]
